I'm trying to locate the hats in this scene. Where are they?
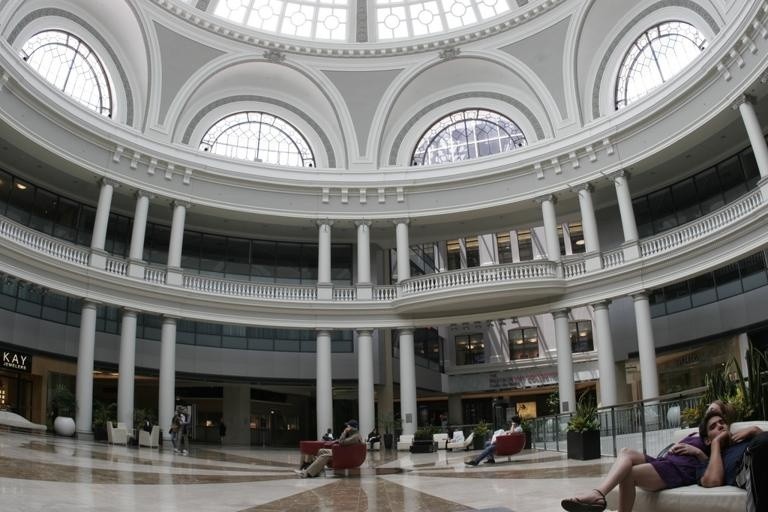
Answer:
[179,407,188,413]
[348,419,359,427]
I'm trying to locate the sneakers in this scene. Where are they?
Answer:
[464,459,495,466]
[174,448,189,453]
[294,469,308,478]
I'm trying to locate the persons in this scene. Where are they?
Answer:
[559,398,739,511]
[367,427,382,451]
[321,428,337,441]
[174,404,191,455]
[463,414,523,466]
[694,409,767,511]
[169,409,179,449]
[293,418,361,479]
[131,416,153,446]
[219,420,226,445]
[180,413,186,424]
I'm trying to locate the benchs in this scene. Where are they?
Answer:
[605,420,767,512]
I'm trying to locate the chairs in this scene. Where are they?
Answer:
[365,437,381,451]
[300,441,366,478]
[107,421,160,449]
[396,435,415,450]
[432,431,474,451]
[491,432,526,462]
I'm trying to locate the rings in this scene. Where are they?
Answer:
[684,444,688,447]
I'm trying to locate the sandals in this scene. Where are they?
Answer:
[561,489,607,512]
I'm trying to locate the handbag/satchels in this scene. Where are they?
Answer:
[300,461,320,477]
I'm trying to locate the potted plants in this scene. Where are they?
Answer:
[520,416,531,449]
[567,388,600,461]
[54,383,76,436]
[473,418,487,449]
